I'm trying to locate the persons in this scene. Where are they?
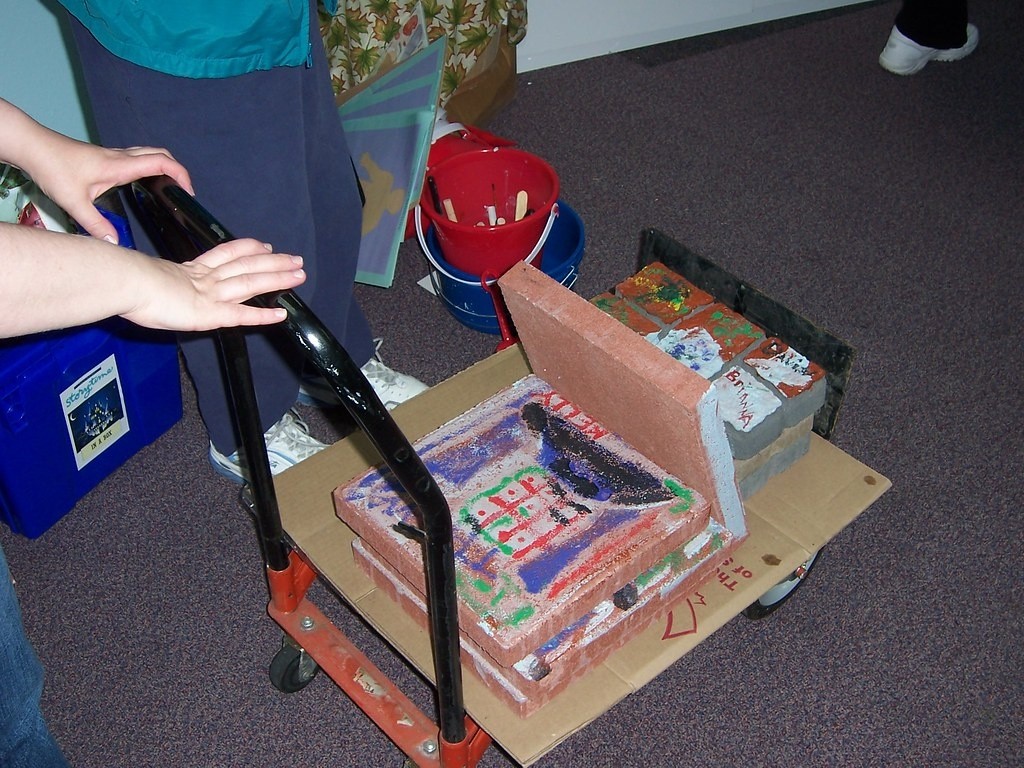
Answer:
[877,0,979,77]
[62,0,430,482]
[1,99,306,768]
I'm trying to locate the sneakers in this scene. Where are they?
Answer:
[879,22,979,77]
[208,407,331,485]
[297,337,430,424]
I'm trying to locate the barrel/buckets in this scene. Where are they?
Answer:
[414,148,561,288]
[426,200,585,334]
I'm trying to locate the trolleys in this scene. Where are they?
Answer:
[117,164,852,768]
